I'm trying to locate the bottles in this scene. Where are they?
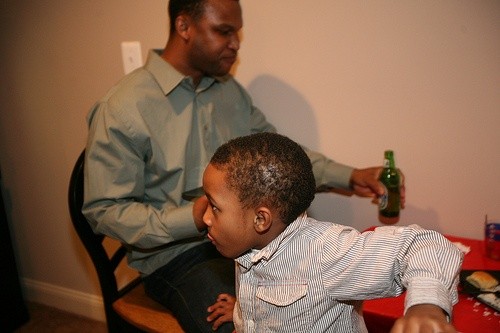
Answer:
[378,151,401,217]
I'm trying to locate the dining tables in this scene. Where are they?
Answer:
[361,226,500,333]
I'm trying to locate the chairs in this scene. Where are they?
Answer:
[67,147,197,333]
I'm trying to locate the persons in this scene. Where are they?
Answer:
[82,0,406,333]
[202,132,463,333]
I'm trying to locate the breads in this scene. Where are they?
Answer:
[466,271,499,289]
